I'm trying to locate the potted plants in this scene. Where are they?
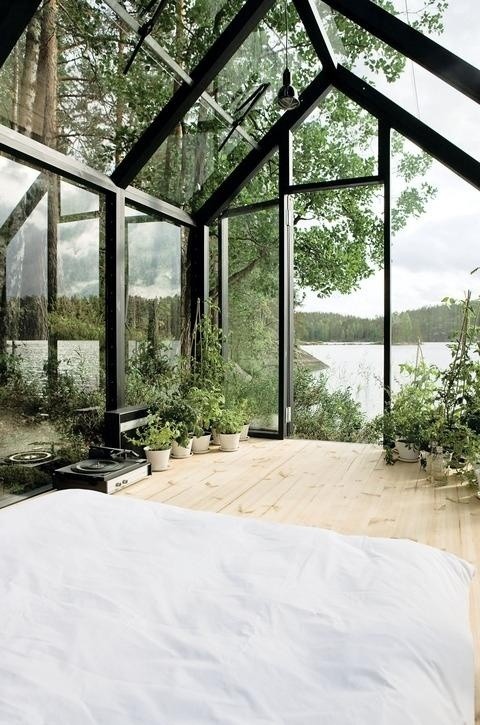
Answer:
[362,289,479,501]
[124,294,277,472]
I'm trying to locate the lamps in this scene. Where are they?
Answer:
[275,1,301,113]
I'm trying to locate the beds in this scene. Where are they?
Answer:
[1,486,477,724]
